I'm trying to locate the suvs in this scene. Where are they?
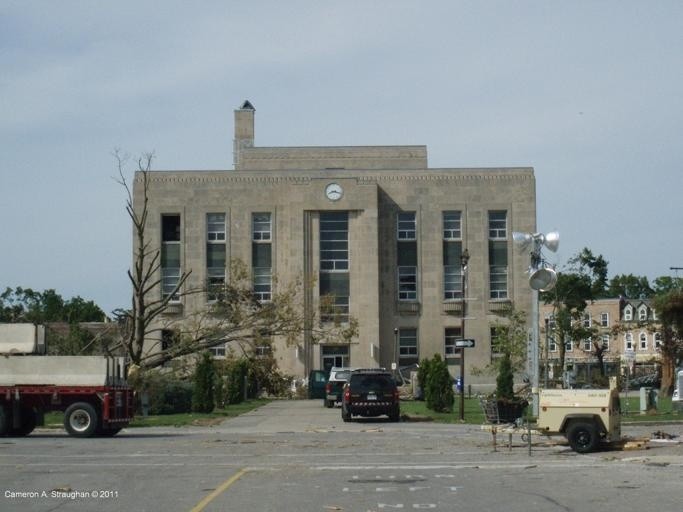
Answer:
[309,366,352,407]
[341,368,400,422]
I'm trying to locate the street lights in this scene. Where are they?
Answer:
[544,313,548,388]
[394,328,398,385]
[459,249,469,418]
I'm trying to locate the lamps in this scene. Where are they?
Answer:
[370,343,377,358]
[295,347,301,357]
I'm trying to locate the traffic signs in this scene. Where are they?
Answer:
[456,339,475,348]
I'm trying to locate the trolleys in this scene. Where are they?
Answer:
[478,397,529,443]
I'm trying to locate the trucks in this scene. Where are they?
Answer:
[0,353,133,436]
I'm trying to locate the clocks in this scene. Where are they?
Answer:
[325,182,345,202]
